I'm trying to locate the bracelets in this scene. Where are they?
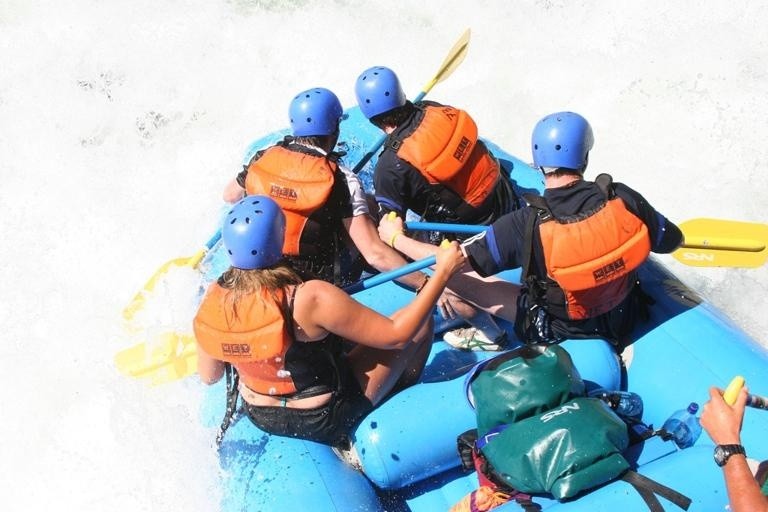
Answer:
[416,274,430,296]
[390,230,403,248]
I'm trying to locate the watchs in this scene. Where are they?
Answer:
[714,444,747,467]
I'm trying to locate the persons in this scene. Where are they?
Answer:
[222,88,457,320]
[699,386,768,512]
[354,65,527,264]
[376,111,683,352]
[193,194,466,447]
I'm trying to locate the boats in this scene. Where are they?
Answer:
[204,101,768,510]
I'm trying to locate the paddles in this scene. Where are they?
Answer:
[353,27,471,173]
[113,239,450,380]
[388,211,768,268]
[116,228,222,329]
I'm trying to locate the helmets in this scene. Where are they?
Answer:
[223,195,285,270]
[355,66,406,119]
[288,88,342,136]
[532,112,593,169]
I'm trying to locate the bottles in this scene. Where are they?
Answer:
[659,402,698,448]
[612,391,643,417]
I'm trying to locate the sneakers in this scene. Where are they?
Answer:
[443,328,508,351]
